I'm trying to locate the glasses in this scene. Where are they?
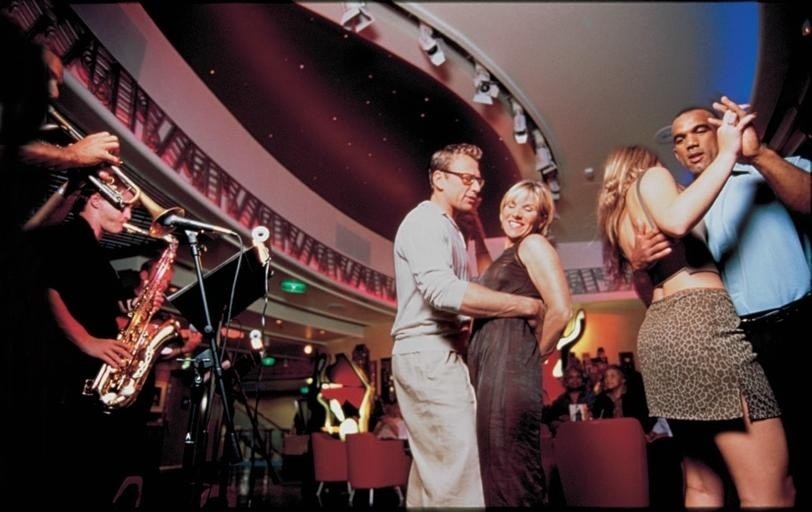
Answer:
[440,169,484,187]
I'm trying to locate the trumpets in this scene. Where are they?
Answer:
[48,106,185,238]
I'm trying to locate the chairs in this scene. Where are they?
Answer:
[311,430,411,507]
[551,417,648,507]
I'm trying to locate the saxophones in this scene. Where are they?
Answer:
[90,222,183,411]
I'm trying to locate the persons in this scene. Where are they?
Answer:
[596,98,811,508]
[0,39,203,369]
[393,142,575,508]
[554,347,670,443]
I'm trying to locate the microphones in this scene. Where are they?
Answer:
[159,214,229,238]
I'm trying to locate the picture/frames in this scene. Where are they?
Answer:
[150,380,167,412]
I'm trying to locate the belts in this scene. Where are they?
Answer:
[740,306,798,330]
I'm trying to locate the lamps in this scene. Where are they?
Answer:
[341,1,562,206]
